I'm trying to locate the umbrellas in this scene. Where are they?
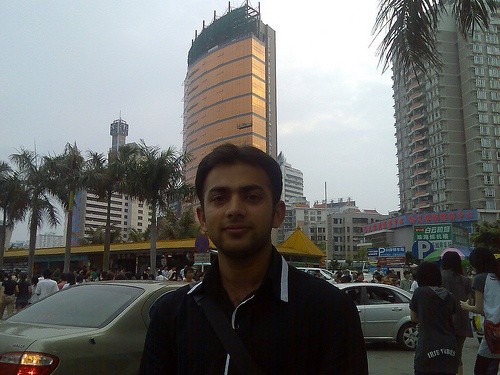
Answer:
[423,247,471,263]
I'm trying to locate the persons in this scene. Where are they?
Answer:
[409,246,500,375]
[296,265,491,342]
[139,143,368,375]
[0,265,204,321]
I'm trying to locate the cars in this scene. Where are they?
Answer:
[297,267,335,282]
[0,280,201,375]
[335,284,419,351]
